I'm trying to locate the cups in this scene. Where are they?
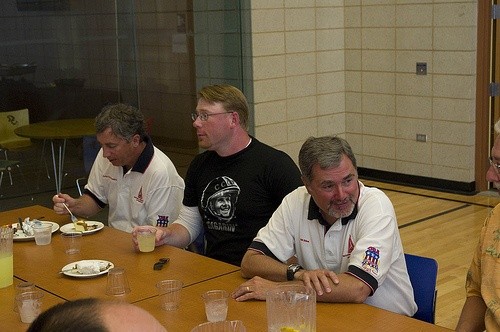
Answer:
[105,268,130,296]
[14,292,44,324]
[31,223,53,246]
[135,227,157,253]
[14,282,41,312]
[160,280,180,310]
[201,289,229,322]
[266,284,316,332]
[60,231,83,255]
[0,227,14,288]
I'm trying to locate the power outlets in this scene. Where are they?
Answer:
[416,133,427,142]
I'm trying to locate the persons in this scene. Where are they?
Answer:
[132,83,304,267]
[26,297,169,332]
[455,117,500,332]
[52,103,186,234]
[232,136,419,319]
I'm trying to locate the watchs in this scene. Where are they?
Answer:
[287,264,305,281]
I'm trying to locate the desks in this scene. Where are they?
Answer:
[13,119,103,193]
[0,204,450,332]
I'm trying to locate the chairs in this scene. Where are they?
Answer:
[63,138,102,218]
[0,108,34,186]
[401,251,443,327]
[0,147,34,201]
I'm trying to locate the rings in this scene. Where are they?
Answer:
[247,287,249,293]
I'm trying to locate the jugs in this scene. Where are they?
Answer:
[190,320,247,332]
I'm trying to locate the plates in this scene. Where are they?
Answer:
[61,260,114,279]
[2,221,59,242]
[60,221,104,235]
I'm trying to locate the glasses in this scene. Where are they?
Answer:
[488,159,500,169]
[191,111,233,121]
